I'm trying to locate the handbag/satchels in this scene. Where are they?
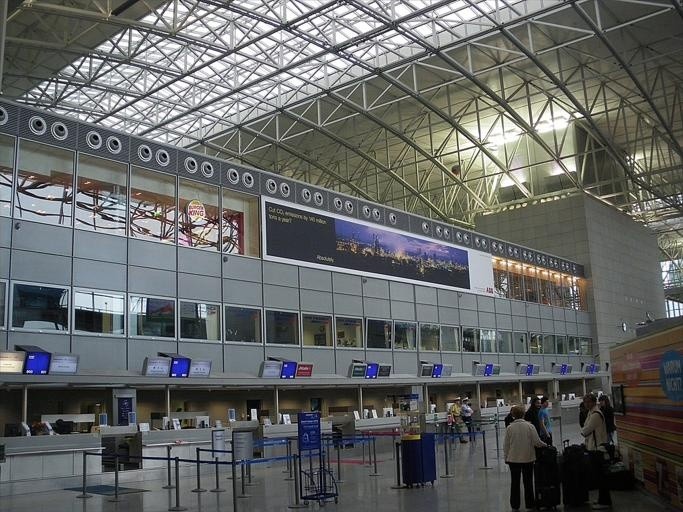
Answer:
[541,446,557,463]
[568,444,586,457]
[597,443,614,462]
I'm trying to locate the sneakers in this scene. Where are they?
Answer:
[591,499,610,510]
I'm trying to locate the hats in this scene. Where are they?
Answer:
[462,397,468,402]
[454,397,460,401]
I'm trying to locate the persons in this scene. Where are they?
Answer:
[502,392,616,512]
[461,397,476,442]
[448,397,468,443]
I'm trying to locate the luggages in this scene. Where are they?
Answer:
[534,445,561,509]
[562,440,589,505]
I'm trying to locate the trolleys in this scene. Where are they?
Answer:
[297,412,339,506]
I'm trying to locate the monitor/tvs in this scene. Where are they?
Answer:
[568,393,575,401]
[4,423,22,437]
[496,399,505,407]
[382,408,394,417]
[195,415,209,428]
[537,395,543,400]
[162,416,170,430]
[446,403,455,412]
[56,421,73,434]
[598,390,604,398]
